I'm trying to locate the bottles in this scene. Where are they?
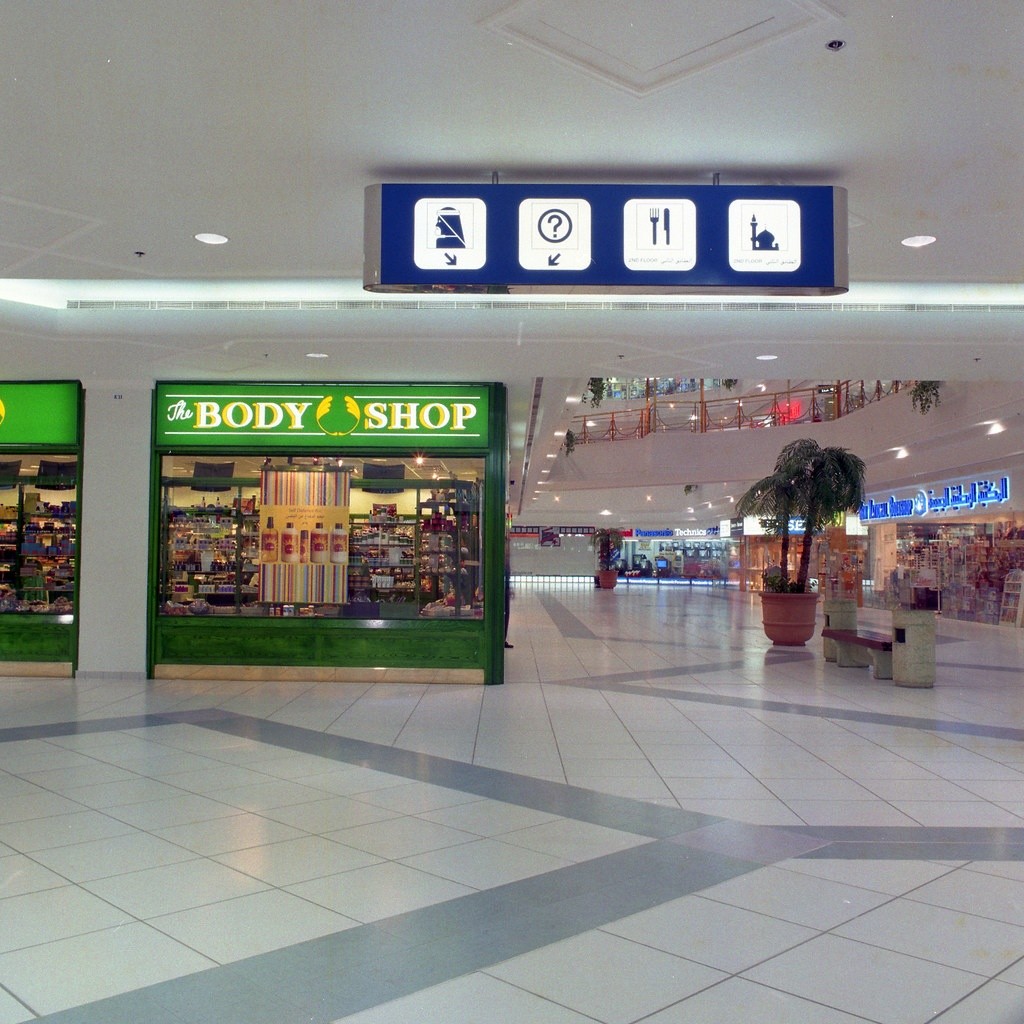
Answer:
[201,496,205,507]
[330,523,347,563]
[281,522,298,563]
[310,523,328,563]
[261,517,278,562]
[300,530,308,563]
[216,497,221,508]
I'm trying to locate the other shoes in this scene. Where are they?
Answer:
[504,641,513,648]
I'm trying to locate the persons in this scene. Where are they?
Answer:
[505,588,515,648]
[442,547,469,606]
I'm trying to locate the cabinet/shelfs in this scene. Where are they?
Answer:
[2,510,74,593]
[168,509,260,603]
[350,521,417,603]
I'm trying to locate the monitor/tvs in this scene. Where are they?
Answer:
[362,463,404,493]
[0,461,23,490]
[35,460,77,491]
[191,461,233,492]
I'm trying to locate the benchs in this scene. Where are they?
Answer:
[821,628,892,651]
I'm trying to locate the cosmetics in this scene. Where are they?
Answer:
[0,493,76,590]
[167,495,415,617]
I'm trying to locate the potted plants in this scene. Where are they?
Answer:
[589,528,624,589]
[736,438,865,647]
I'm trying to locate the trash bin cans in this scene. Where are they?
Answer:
[821,599,858,662]
[891,609,938,689]
